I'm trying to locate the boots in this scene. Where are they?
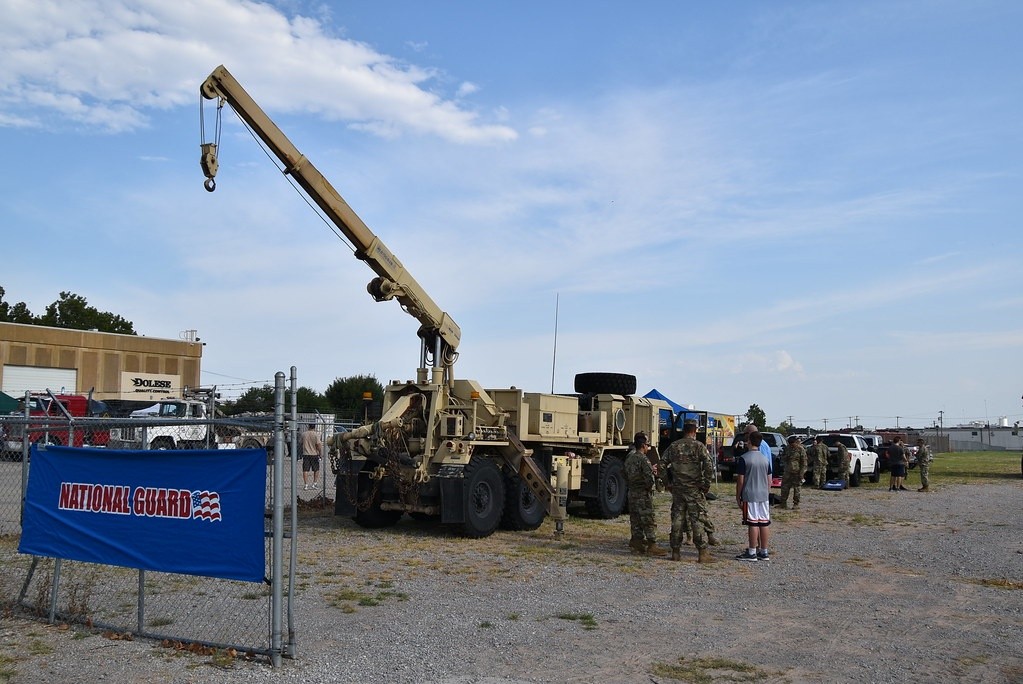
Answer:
[672,546,680,561]
[707,533,719,545]
[647,543,668,556]
[685,533,693,545]
[698,548,718,563]
[629,538,648,554]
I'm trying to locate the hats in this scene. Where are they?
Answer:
[684,419,697,424]
[916,438,926,444]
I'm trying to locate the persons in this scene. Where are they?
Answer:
[812,437,829,489]
[811,439,817,448]
[299,423,323,491]
[796,438,807,451]
[833,439,850,489]
[886,436,910,491]
[743,425,772,551]
[914,438,930,492]
[779,436,807,510]
[624,419,719,564]
[735,431,770,562]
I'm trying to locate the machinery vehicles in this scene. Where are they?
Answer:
[198,64,674,538]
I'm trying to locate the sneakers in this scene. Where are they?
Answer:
[757,552,769,560]
[735,548,758,561]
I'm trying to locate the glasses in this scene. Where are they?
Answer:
[645,444,649,447]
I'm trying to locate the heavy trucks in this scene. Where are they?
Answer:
[109,398,336,465]
[3,392,130,451]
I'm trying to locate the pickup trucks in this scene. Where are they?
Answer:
[803,433,881,487]
[864,436,919,469]
[717,431,789,482]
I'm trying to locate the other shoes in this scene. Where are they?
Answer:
[811,485,819,489]
[304,484,309,491]
[918,486,929,492]
[889,484,907,492]
[311,483,318,490]
